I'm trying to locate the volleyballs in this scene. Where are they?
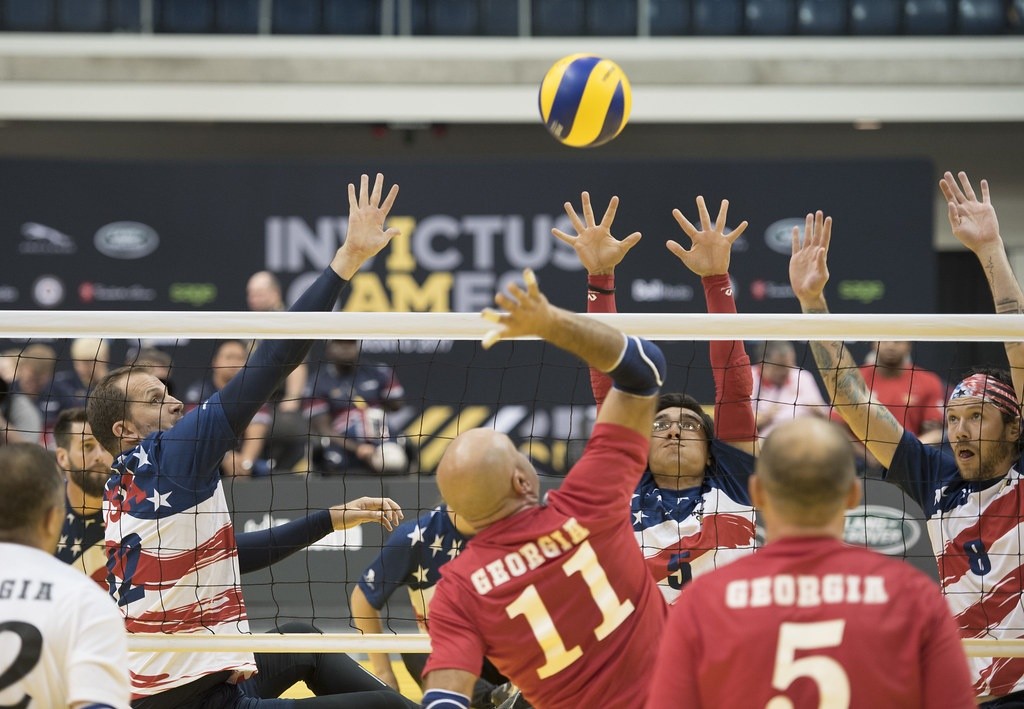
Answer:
[539,51,633,148]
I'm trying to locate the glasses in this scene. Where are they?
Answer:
[651,420,707,437]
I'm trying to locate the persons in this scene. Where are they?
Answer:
[1,440,130,708]
[53,410,423,709]
[789,171,1024,708]
[95,173,403,707]
[648,415,975,707]
[423,267,668,707]
[549,191,755,602]
[0,266,947,480]
[345,468,549,705]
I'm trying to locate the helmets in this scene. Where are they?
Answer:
[371,441,409,475]
